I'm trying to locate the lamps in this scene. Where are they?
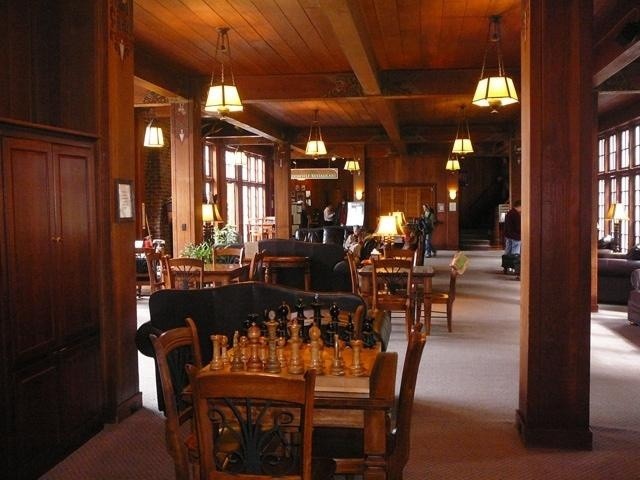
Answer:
[373,211,408,260]
[205,25,244,119]
[142,118,164,148]
[344,145,361,173]
[202,203,224,246]
[305,109,328,159]
[452,104,474,157]
[471,14,519,114]
[607,203,631,252]
[446,140,460,172]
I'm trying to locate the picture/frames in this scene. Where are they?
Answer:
[113,176,135,225]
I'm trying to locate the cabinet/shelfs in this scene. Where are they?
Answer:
[0,119,101,480]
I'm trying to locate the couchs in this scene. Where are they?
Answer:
[257,239,354,292]
[135,281,392,418]
[295,225,353,245]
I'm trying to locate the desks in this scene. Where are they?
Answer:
[260,256,311,292]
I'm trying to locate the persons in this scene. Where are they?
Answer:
[423,204,437,258]
[346,225,374,268]
[504,200,521,275]
[324,203,335,226]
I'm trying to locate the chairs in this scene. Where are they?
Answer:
[412,253,466,336]
[387,322,426,479]
[149,317,202,479]
[186,363,317,479]
[135,247,252,299]
[347,235,434,336]
[597,244,640,307]
[246,216,276,241]
[627,268,640,327]
